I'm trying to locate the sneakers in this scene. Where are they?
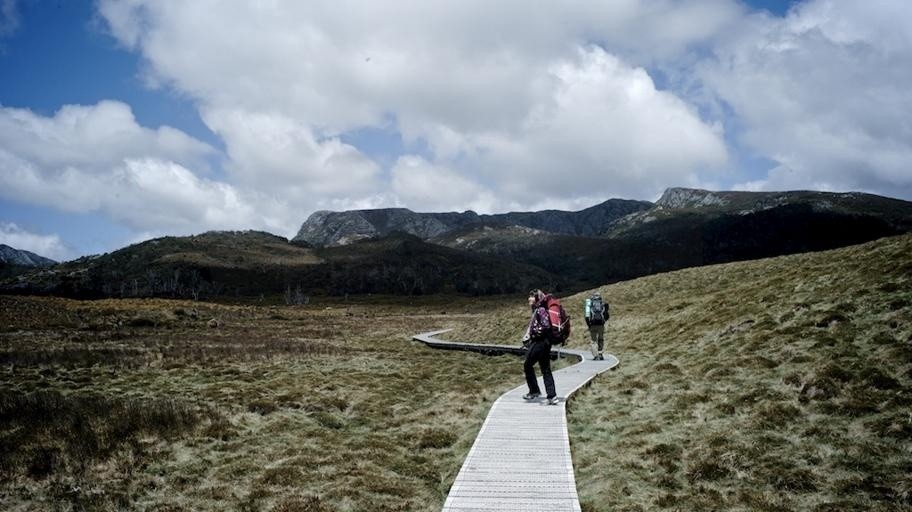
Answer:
[593,356,598,360]
[599,353,603,360]
[522,391,542,401]
[540,395,559,406]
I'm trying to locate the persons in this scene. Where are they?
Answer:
[521,288,560,405]
[584,292,608,360]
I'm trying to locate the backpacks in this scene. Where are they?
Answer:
[544,293,570,344]
[589,292,606,324]
